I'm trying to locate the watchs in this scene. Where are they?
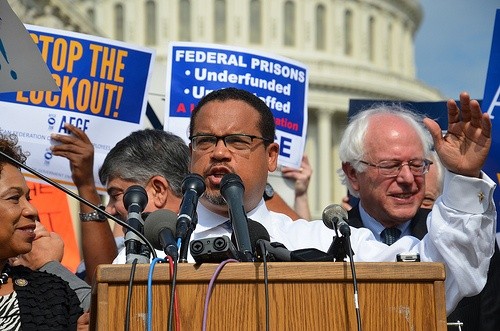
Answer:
[263,183,274,201]
[79,205,107,221]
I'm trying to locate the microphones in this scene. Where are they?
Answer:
[124,185,149,261]
[219,174,254,262]
[175,172,206,244]
[322,203,351,237]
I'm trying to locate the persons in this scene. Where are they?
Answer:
[0,138,90,331]
[263,183,301,221]
[281,154,313,222]
[339,108,500,331]
[113,87,496,316]
[420,154,443,212]
[23,129,190,312]
[51,122,117,284]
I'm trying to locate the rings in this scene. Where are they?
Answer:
[300,168,303,173]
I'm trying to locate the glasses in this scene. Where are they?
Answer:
[353,158,433,178]
[189,133,272,154]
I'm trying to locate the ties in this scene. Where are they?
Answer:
[380,227,402,246]
